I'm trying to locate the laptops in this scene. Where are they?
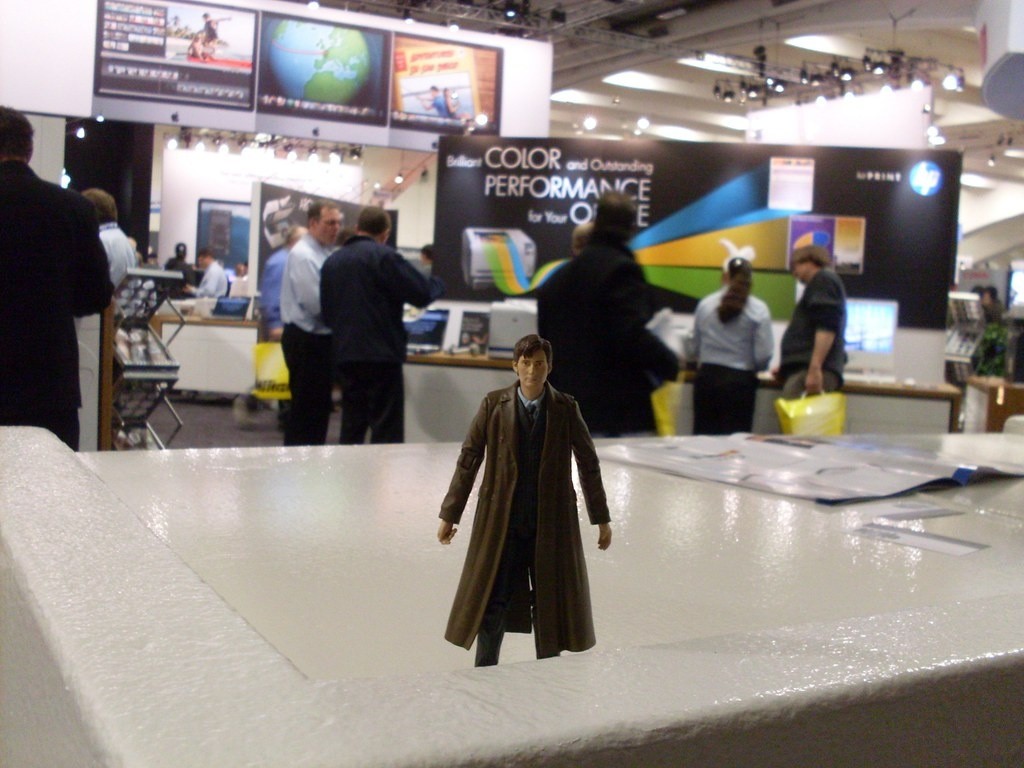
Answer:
[403,310,450,354]
[843,298,899,371]
[202,295,251,321]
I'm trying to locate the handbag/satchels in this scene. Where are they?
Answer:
[651,371,695,438]
[774,388,846,438]
[252,338,293,401]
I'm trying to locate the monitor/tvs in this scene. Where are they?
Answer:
[90,0,506,153]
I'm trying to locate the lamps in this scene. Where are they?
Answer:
[505,2,518,18]
[402,8,414,22]
[179,127,363,161]
[988,135,1013,168]
[712,49,964,113]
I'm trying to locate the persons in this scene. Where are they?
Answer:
[317,206,448,445]
[165,244,193,271]
[679,257,776,436]
[80,188,137,286]
[186,11,233,62]
[542,190,682,440]
[773,244,850,436]
[971,285,1006,324]
[0,105,115,452]
[254,200,357,446]
[422,86,460,119]
[183,248,248,298]
[438,334,611,667]
